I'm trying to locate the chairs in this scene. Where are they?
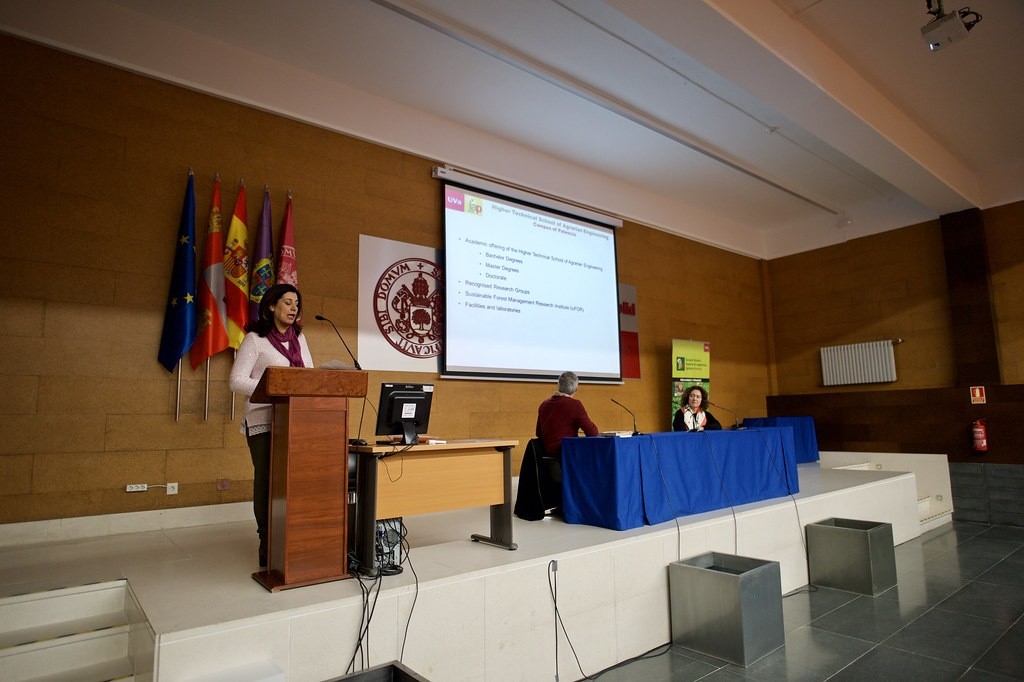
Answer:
[531,439,562,518]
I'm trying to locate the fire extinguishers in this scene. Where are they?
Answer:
[972,418,988,450]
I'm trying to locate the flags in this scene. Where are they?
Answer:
[223,187,248,348]
[275,195,301,323]
[189,178,229,370]
[247,191,277,322]
[157,174,196,374]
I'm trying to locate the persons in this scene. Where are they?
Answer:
[537,372,598,466]
[673,386,722,431]
[229,285,315,568]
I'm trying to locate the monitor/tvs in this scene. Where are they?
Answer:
[375,383,434,445]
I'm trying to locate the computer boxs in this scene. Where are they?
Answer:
[373,517,403,573]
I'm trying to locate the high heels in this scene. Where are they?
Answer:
[259,542,267,567]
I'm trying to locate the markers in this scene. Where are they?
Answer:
[426,440,446,444]
[620,435,631,437]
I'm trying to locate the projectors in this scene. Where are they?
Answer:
[921,9,970,51]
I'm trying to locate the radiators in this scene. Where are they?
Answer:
[821,338,905,386]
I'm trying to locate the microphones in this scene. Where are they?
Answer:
[709,402,746,431]
[315,315,362,371]
[670,400,698,432]
[611,398,642,436]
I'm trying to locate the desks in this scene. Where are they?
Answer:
[742,416,820,464]
[562,425,800,532]
[347,439,521,576]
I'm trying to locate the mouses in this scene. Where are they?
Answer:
[352,439,368,446]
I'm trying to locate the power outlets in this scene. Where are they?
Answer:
[126,484,147,491]
[167,483,179,495]
